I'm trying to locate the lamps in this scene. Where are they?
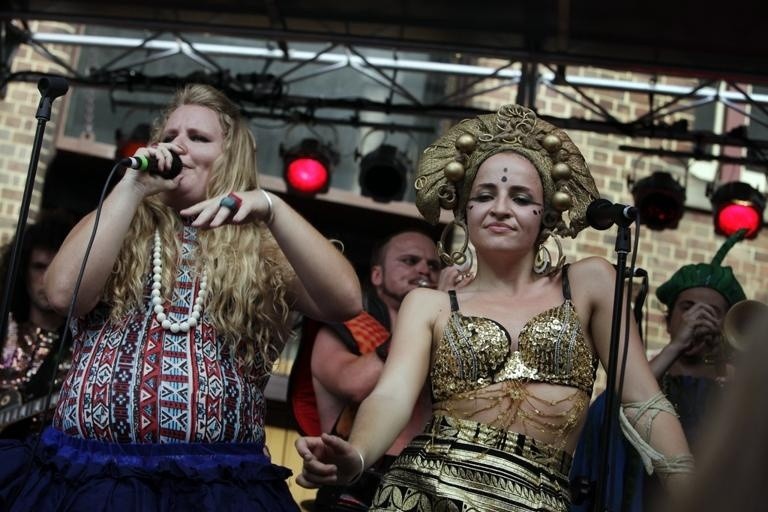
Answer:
[628,174,768,241]
[279,140,411,204]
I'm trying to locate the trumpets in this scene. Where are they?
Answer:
[703,300,767,364]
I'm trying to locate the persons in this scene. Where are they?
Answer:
[2,220,92,410]
[571,263,753,512]
[309,231,473,512]
[2,84,366,511]
[296,103,707,512]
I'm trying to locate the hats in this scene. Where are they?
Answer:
[656,229,748,307]
[414,106,602,279]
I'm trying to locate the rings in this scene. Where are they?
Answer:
[222,191,241,213]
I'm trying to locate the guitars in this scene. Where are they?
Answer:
[0,391,59,431]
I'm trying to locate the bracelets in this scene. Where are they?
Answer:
[342,442,365,487]
[252,188,274,230]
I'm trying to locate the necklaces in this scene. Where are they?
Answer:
[152,220,216,333]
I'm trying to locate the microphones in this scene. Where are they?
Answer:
[586,197,638,231]
[121,149,184,180]
